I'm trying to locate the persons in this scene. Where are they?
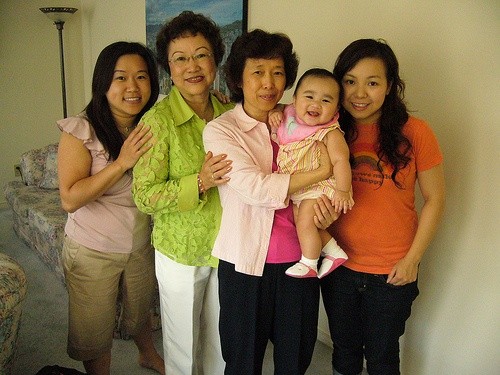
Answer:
[268,67,355,278]
[319,39,445,375]
[56,41,166,375]
[202,28,340,375]
[132,10,238,375]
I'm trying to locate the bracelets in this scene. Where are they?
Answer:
[197,173,207,194]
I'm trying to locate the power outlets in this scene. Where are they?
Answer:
[14,163,21,176]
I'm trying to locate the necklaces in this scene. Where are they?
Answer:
[118,122,133,132]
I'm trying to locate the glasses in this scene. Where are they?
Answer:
[167,51,214,65]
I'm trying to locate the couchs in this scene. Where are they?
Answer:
[4,143,163,339]
[0,253,28,375]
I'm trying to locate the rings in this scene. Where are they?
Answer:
[211,174,216,180]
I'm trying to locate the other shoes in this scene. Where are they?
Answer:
[285,260,318,277]
[138,353,165,375]
[317,249,348,279]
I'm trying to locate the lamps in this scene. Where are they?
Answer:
[39,8,78,122]
